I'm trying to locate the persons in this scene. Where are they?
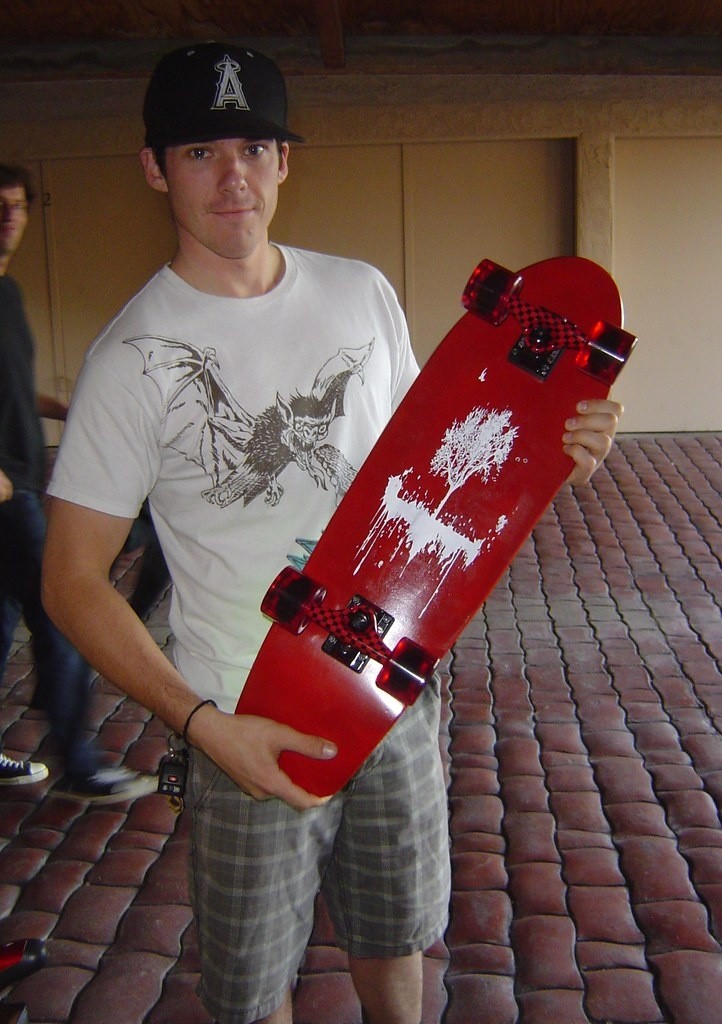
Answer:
[1,156,159,806]
[42,47,625,1024]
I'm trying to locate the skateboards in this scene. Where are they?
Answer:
[232,252,640,798]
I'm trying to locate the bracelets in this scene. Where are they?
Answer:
[183,699,218,741]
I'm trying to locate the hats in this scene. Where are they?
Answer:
[142,41,306,147]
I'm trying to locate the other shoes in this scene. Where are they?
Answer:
[0,752,49,785]
[44,765,158,805]
